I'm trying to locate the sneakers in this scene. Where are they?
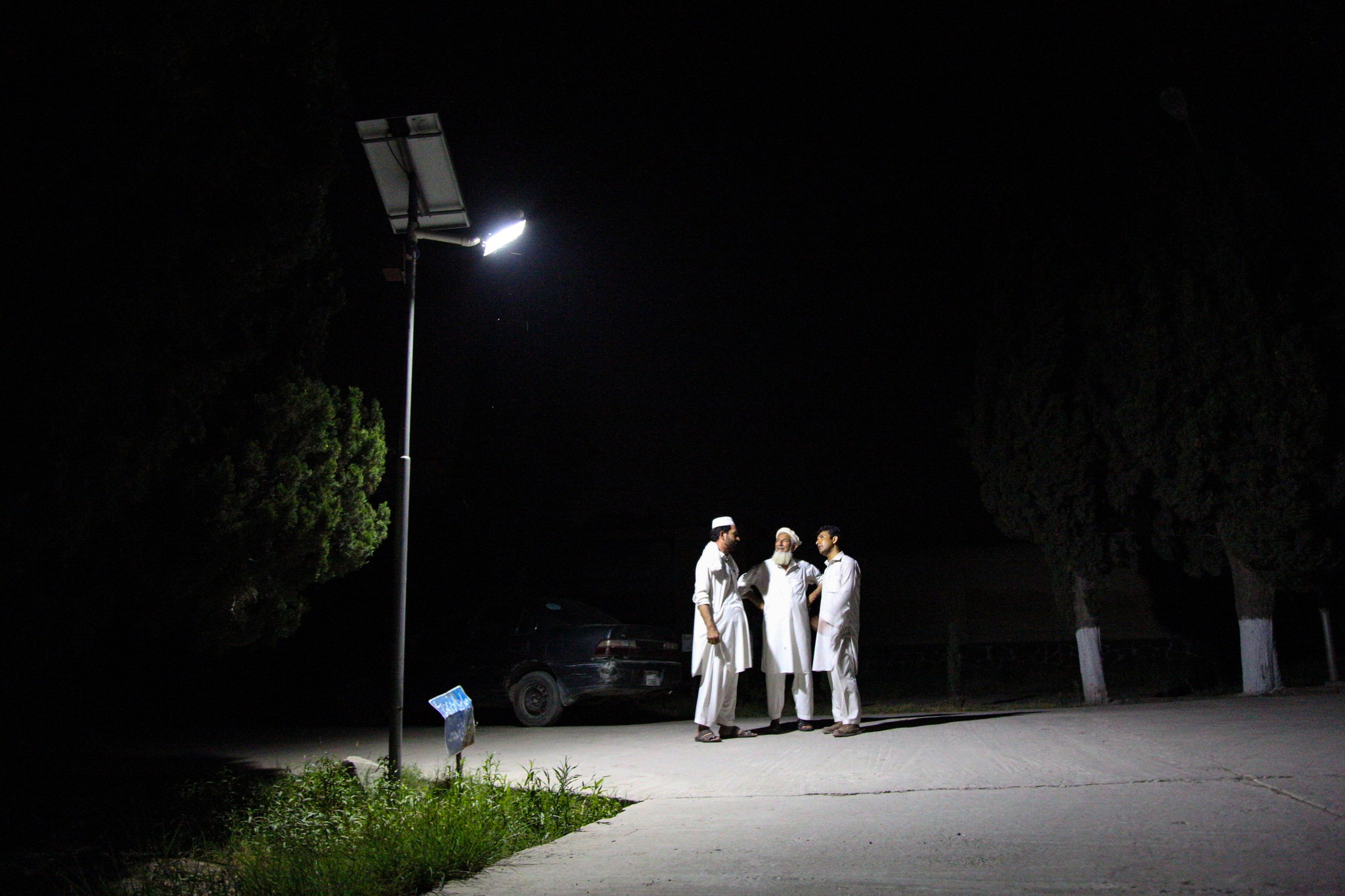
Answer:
[822,721,843,733]
[832,724,862,736]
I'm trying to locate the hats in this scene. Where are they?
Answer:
[712,516,735,528]
[776,527,800,546]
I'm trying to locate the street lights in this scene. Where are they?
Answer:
[387,207,532,794]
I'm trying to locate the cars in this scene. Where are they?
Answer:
[483,600,686,728]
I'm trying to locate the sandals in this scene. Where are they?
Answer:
[796,719,813,731]
[768,721,781,733]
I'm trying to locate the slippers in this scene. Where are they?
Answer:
[720,726,758,737]
[695,729,722,742]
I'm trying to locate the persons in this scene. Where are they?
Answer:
[691,516,756,744]
[736,529,824,735]
[808,526,861,738]
